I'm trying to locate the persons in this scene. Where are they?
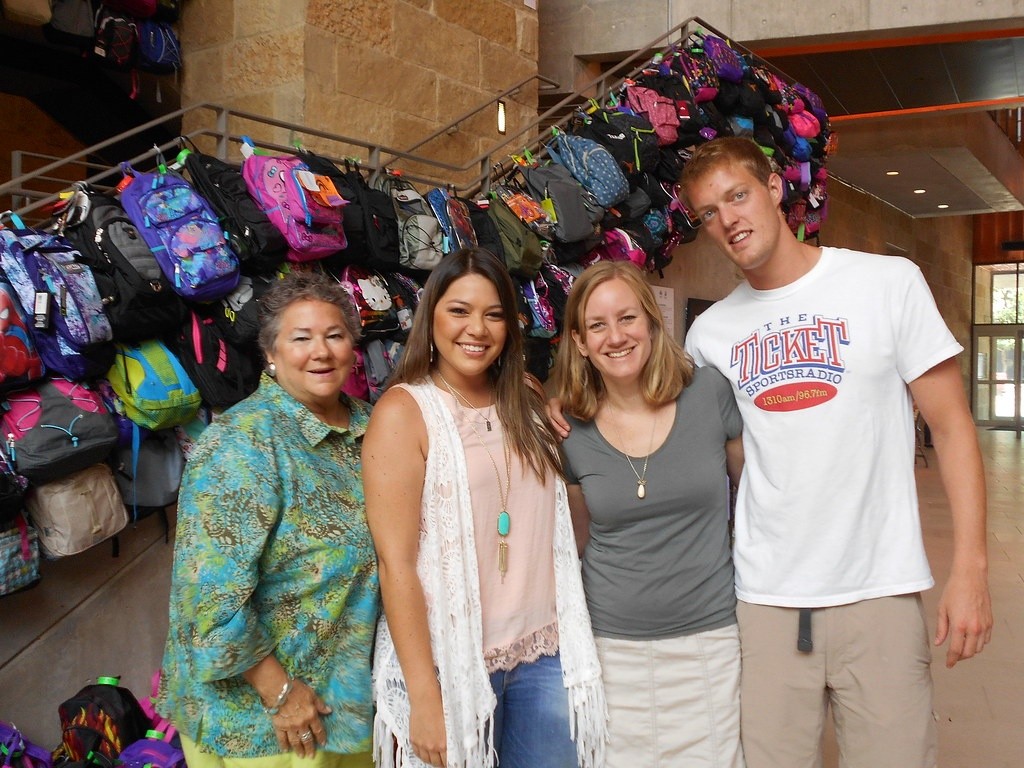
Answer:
[545,137,993,768]
[360,244,607,768]
[153,275,386,768]
[535,255,748,768]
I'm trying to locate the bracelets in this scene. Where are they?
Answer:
[259,665,294,715]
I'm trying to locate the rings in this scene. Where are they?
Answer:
[301,731,313,743]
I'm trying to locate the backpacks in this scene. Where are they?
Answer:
[100,425,185,507]
[0,273,46,399]
[240,134,352,265]
[156,303,265,412]
[177,132,288,272]
[22,460,130,561]
[278,28,839,408]
[193,253,281,355]
[100,332,204,432]
[0,211,116,382]
[114,158,242,309]
[0,502,43,597]
[172,403,225,461]
[0,667,187,767]
[0,371,120,488]
[41,180,192,349]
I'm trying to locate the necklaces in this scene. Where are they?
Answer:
[436,369,511,585]
[448,384,492,432]
[605,395,656,498]
